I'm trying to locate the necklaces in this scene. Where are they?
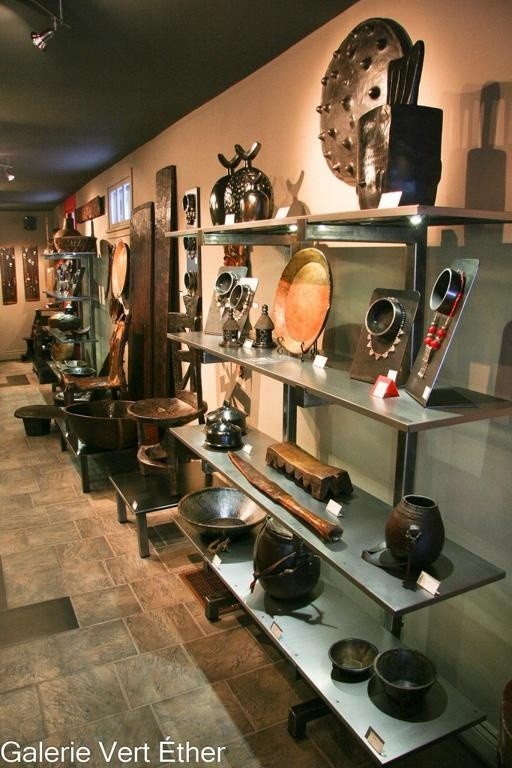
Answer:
[214,270,240,321]
[366,295,407,360]
[230,283,253,322]
[414,267,466,382]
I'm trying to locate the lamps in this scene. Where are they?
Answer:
[4,167,14,182]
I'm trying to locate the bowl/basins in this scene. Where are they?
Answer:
[66,400,137,450]
[204,400,248,445]
[179,487,268,532]
[328,638,379,672]
[374,648,437,699]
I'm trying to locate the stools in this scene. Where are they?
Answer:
[13,404,60,436]
[109,461,232,560]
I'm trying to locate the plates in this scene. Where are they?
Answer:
[111,241,129,296]
[272,246,334,355]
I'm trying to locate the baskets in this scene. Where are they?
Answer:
[55,236,97,252]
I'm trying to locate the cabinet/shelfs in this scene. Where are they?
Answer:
[166,204,510,768]
[40,248,98,396]
